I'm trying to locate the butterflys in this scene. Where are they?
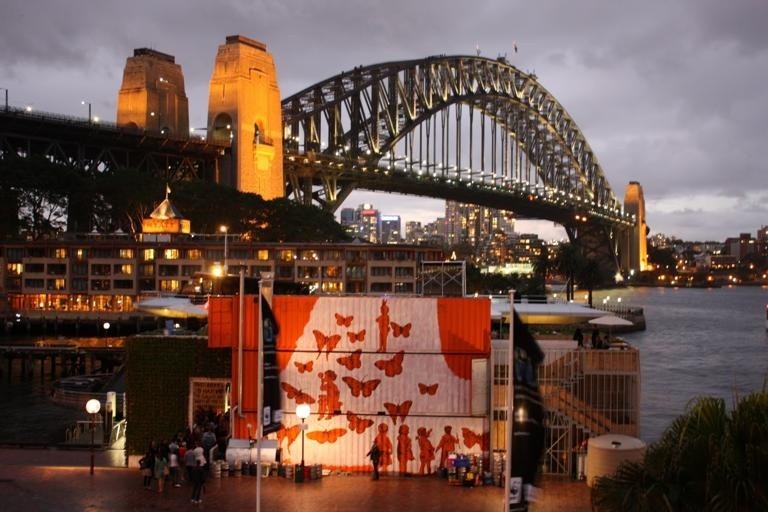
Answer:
[418,383,438,395]
[390,322,412,337]
[374,350,405,377]
[306,428,347,444]
[347,411,374,433]
[384,400,413,425]
[462,428,490,451]
[294,360,313,373]
[280,382,315,404]
[276,424,301,453]
[311,311,366,371]
[342,374,381,398]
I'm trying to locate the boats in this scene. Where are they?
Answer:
[130,271,310,320]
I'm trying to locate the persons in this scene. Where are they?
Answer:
[366,440,380,482]
[573,324,610,350]
[139,409,233,504]
[239,208,288,244]
[496,316,506,338]
[21,225,49,241]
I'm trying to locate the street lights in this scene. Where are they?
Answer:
[220,223,232,277]
[295,400,310,478]
[84,396,103,476]
[150,111,162,135]
[546,290,628,323]
[101,318,115,372]
[0,87,12,113]
[81,101,93,126]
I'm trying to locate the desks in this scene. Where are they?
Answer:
[608,342,629,350]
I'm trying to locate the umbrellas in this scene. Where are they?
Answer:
[588,313,634,345]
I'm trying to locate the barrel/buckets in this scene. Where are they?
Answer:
[213,459,321,485]
[446,454,507,486]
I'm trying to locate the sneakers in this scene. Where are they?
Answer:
[191,498,203,504]
